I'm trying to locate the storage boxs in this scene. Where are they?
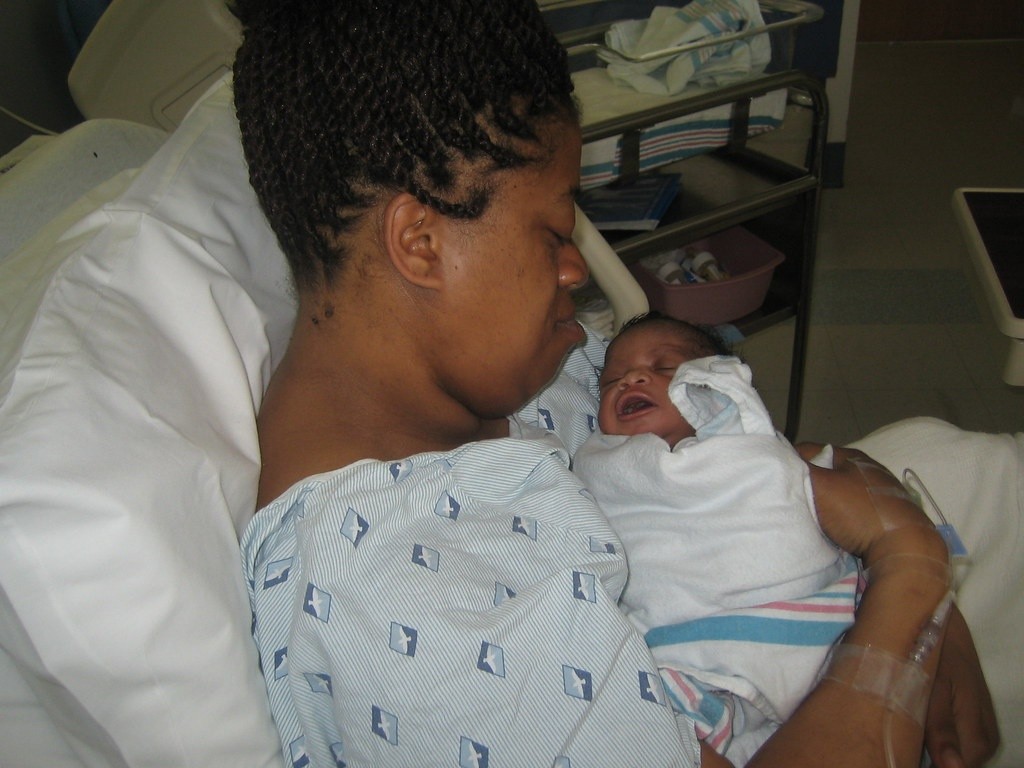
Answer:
[629,223,786,325]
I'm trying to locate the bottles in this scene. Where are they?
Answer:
[685,246,733,284]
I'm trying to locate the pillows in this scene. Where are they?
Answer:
[0,71,300,768]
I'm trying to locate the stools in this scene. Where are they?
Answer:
[950,188,1024,387]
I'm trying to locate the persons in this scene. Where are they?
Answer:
[570,311,865,768]
[231,0,1001,768]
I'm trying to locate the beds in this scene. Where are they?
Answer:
[0,116,1024,768]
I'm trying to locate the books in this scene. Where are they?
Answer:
[577,171,684,230]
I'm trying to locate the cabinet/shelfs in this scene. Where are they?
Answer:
[582,68,829,445]
